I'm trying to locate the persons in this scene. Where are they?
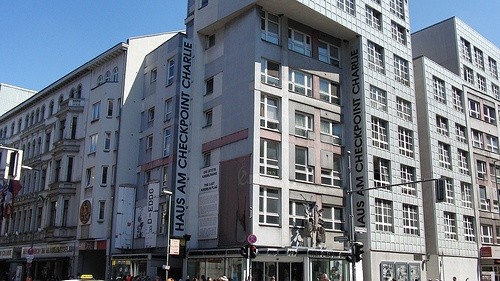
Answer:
[0,271,56,281]
[272,274,275,281]
[453,277,456,281]
[321,273,330,281]
[168,275,228,281]
[113,273,160,281]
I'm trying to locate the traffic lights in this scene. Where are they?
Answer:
[355,243,364,262]
[250,246,256,258]
[346,255,352,262]
[241,245,249,259]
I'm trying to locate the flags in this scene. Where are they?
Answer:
[0,180,21,220]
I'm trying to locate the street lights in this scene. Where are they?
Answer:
[162,190,173,281]
